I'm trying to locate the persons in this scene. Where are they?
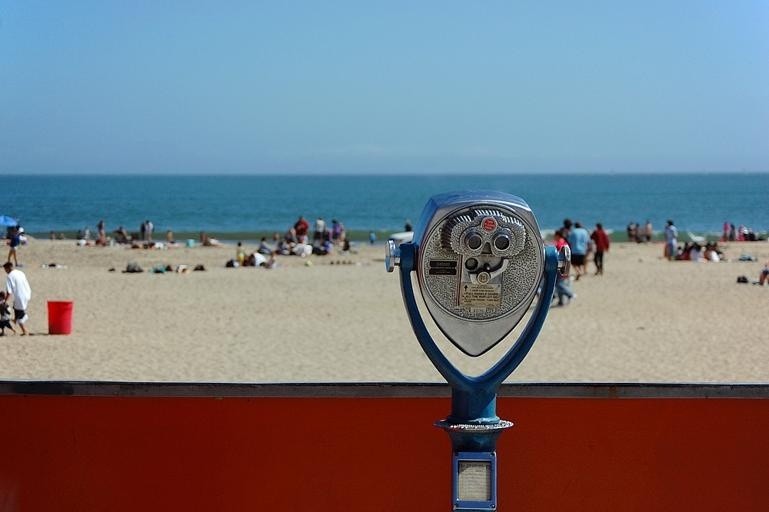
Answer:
[0,291,16,336]
[3,262,32,336]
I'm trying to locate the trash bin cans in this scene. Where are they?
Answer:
[48,300,73,334]
